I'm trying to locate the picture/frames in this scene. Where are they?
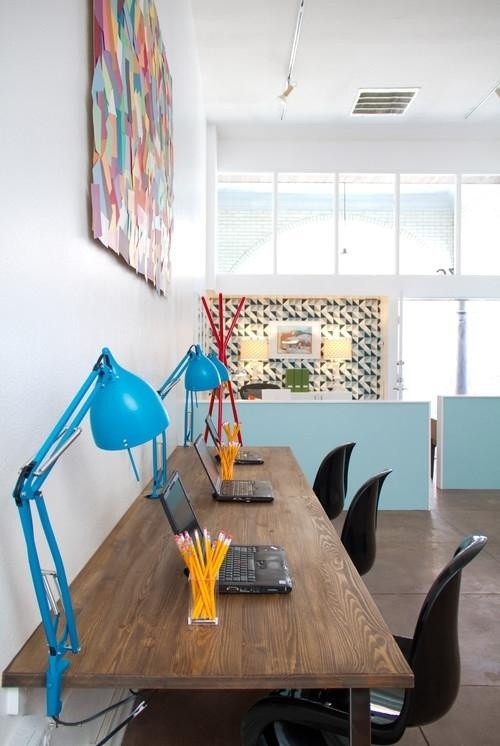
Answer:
[269,321,322,359]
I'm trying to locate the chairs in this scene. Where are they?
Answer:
[313,442,357,519]
[241,529,488,745]
[240,384,280,399]
[341,468,394,574]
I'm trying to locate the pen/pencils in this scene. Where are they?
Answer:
[174,527,233,620]
[215,441,241,481]
[222,421,241,443]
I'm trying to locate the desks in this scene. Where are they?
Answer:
[2,444,417,690]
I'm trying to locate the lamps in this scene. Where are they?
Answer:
[279,74,296,106]
[180,346,230,449]
[12,344,175,746]
[240,337,268,384]
[144,343,223,498]
[323,337,353,391]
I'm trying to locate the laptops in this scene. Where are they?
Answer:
[193,434,274,502]
[159,470,292,594]
[205,413,264,465]
